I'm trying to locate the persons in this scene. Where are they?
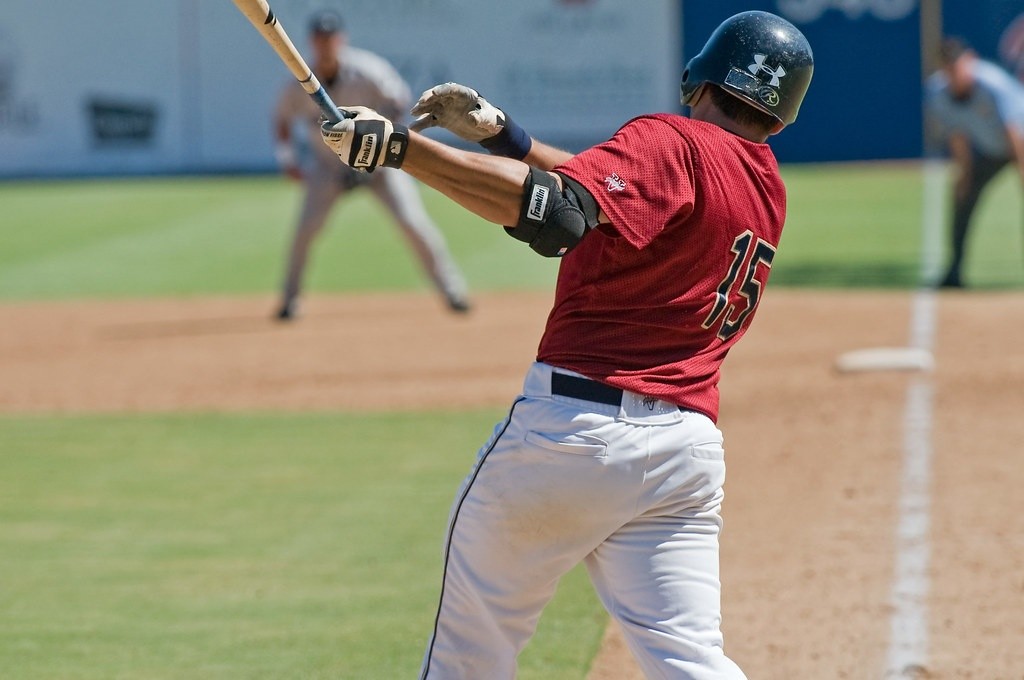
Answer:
[272,9,471,322]
[317,10,813,680]
[922,15,1024,287]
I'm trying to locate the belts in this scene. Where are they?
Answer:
[551,372,690,411]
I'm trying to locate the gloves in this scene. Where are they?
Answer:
[317,106,409,174]
[408,81,532,160]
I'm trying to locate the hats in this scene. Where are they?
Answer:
[935,39,966,63]
[309,8,345,36]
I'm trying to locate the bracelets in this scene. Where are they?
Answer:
[275,144,295,169]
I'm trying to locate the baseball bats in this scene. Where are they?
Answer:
[232,0,344,127]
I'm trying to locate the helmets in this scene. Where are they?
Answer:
[679,12,814,127]
